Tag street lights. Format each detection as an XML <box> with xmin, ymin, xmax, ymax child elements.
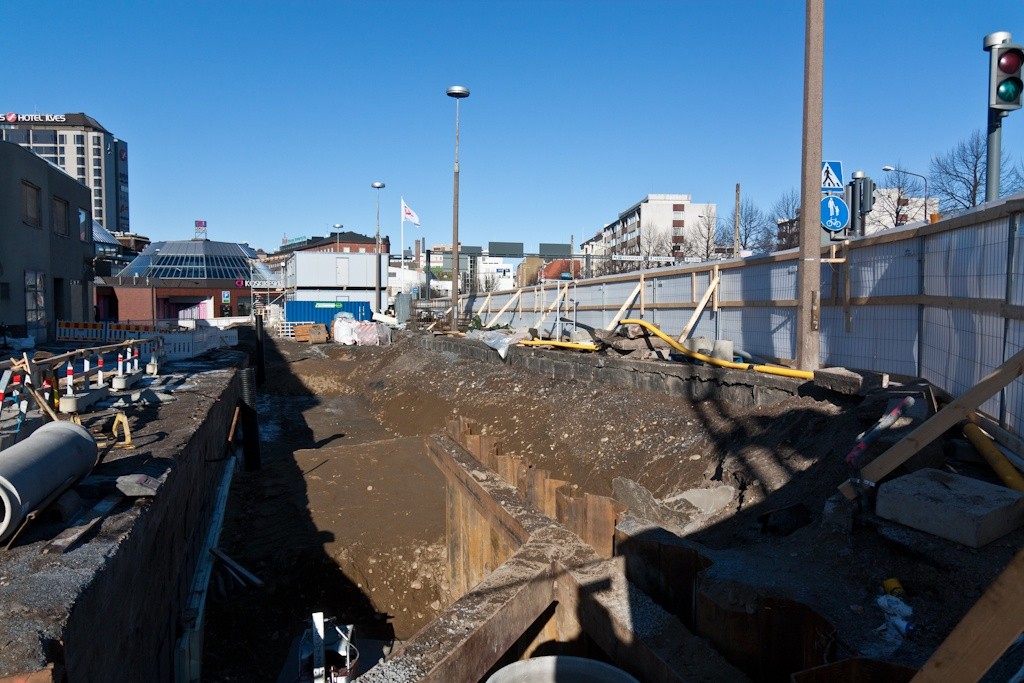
<box><xmin>333</xmin><ymin>223</ymin><xmax>344</xmax><ymax>252</ymax></box>
<box><xmin>445</xmin><ymin>86</ymin><xmax>471</xmax><ymax>333</ymax></box>
<box><xmin>372</xmin><ymin>181</ymin><xmax>385</xmax><ymax>314</ymax></box>
<box><xmin>883</xmin><ymin>166</ymin><xmax>930</xmax><ymax>222</ymax></box>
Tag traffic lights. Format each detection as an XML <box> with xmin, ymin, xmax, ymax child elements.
<box><xmin>990</xmin><ymin>45</ymin><xmax>1024</xmax><ymax>110</ymax></box>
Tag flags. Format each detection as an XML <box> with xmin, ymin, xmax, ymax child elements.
<box><xmin>403</xmin><ymin>200</ymin><xmax>421</xmax><ymax>228</ymax></box>
